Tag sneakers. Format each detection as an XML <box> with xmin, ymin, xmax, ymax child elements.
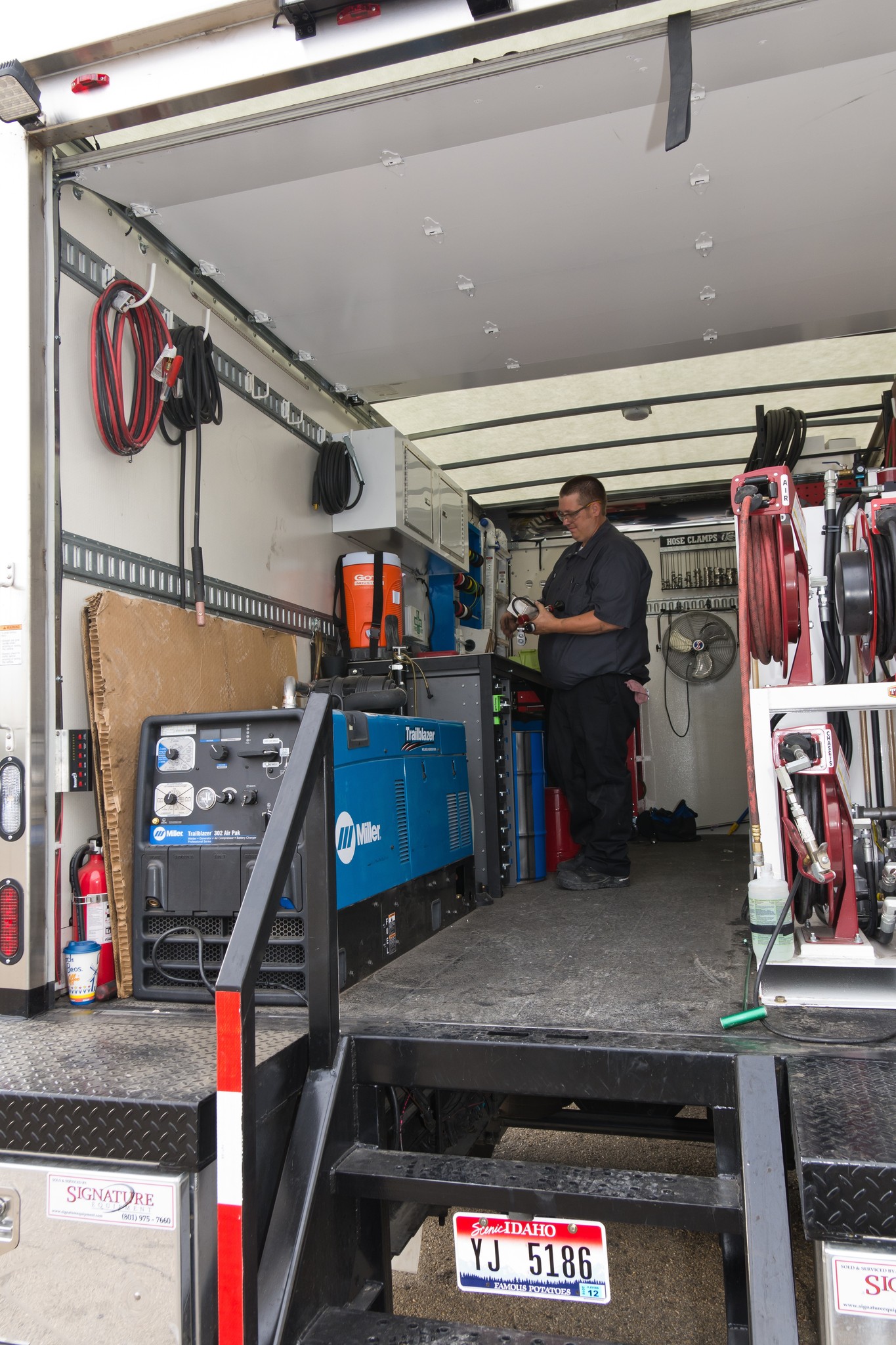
<box><xmin>556</xmin><ymin>852</ymin><xmax>586</xmax><ymax>876</ymax></box>
<box><xmin>555</xmin><ymin>863</ymin><xmax>630</xmax><ymax>891</ymax></box>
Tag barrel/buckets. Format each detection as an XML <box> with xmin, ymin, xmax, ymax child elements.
<box><xmin>334</xmin><ymin>551</ymin><xmax>403</xmax><ymax>648</ymax></box>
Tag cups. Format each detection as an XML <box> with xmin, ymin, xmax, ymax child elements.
<box><xmin>62</xmin><ymin>940</ymin><xmax>102</xmax><ymax>1006</ymax></box>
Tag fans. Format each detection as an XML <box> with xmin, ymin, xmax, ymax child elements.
<box><xmin>660</xmin><ymin>611</ymin><xmax>737</xmax><ymax>682</ymax></box>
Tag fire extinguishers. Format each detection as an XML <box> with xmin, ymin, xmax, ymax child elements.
<box><xmin>69</xmin><ymin>833</ymin><xmax>116</xmax><ymax>987</ymax></box>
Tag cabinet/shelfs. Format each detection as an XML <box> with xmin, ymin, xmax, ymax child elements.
<box><xmin>332</xmin><ymin>426</ymin><xmax>470</xmax><ymax>576</ymax></box>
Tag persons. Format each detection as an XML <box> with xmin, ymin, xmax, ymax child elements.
<box><xmin>500</xmin><ymin>474</ymin><xmax>652</xmax><ymax>890</ymax></box>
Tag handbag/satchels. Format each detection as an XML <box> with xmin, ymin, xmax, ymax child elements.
<box><xmin>636</xmin><ymin>799</ymin><xmax>699</xmax><ymax>843</ymax></box>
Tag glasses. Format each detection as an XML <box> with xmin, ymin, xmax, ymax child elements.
<box><xmin>556</xmin><ymin>501</ymin><xmax>602</xmax><ymax>523</ymax></box>
